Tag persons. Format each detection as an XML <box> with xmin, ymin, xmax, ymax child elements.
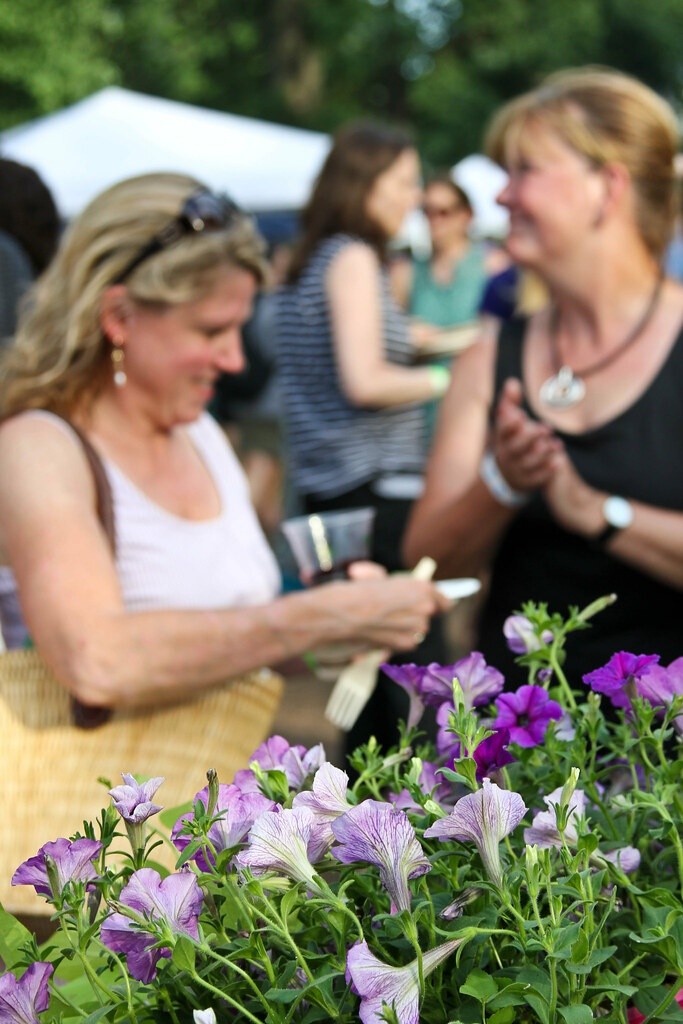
<box><xmin>0</xmin><ymin>171</ymin><xmax>454</xmax><ymax>912</ymax></box>
<box><xmin>273</xmin><ymin>117</ymin><xmax>479</xmax><ymax>576</ymax></box>
<box><xmin>409</xmin><ymin>179</ymin><xmax>505</xmax><ymax>329</ymax></box>
<box><xmin>401</xmin><ymin>63</ymin><xmax>683</xmax><ymax>787</ymax></box>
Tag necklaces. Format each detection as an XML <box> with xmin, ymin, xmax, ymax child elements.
<box><xmin>540</xmin><ymin>269</ymin><xmax>664</xmax><ymax>411</ymax></box>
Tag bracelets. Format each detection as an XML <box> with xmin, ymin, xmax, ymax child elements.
<box><xmin>481</xmin><ymin>457</ymin><xmax>524</xmax><ymax>508</ymax></box>
<box><xmin>429</xmin><ymin>363</ymin><xmax>450</xmax><ymax>399</ymax></box>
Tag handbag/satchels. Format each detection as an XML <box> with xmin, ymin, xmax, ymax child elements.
<box><xmin>0</xmin><ymin>407</ymin><xmax>283</xmax><ymax>918</ymax></box>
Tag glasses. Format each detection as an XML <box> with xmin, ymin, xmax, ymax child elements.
<box><xmin>114</xmin><ymin>189</ymin><xmax>228</xmax><ymax>285</ymax></box>
<box><xmin>421</xmin><ymin>203</ymin><xmax>465</xmax><ymax>218</ymax></box>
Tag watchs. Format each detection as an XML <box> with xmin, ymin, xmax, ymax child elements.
<box><xmin>595</xmin><ymin>497</ymin><xmax>633</xmax><ymax>544</ymax></box>
